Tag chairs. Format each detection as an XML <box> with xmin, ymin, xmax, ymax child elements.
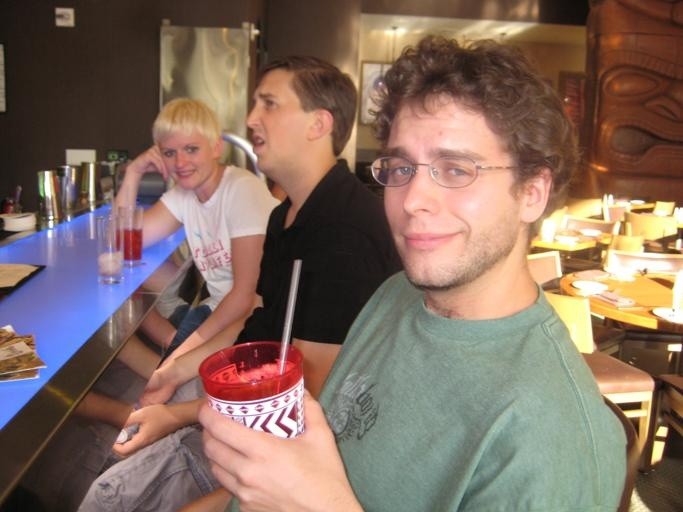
<box><xmin>529</xmin><ymin>198</ymin><xmax>683</xmax><ymax>512</ymax></box>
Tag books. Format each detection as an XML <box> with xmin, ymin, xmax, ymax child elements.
<box><xmin>0</xmin><ymin>324</ymin><xmax>48</xmax><ymax>382</ymax></box>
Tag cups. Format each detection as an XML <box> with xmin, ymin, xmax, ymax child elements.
<box><xmin>97</xmin><ymin>205</ymin><xmax>145</xmax><ymax>286</ymax></box>
<box><xmin>37</xmin><ymin>162</ymin><xmax>99</xmax><ymax>222</ymax></box>
<box><xmin>540</xmin><ymin>219</ymin><xmax>556</xmax><ymax>243</ymax></box>
<box><xmin>199</xmin><ymin>341</ymin><xmax>306</xmax><ymax>439</ymax></box>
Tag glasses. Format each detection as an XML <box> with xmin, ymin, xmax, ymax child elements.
<box><xmin>365</xmin><ymin>153</ymin><xmax>520</xmax><ymax>188</ymax></box>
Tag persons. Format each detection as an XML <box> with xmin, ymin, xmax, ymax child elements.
<box><xmin>76</xmin><ymin>54</ymin><xmax>405</xmax><ymax>511</ymax></box>
<box><xmin>107</xmin><ymin>98</ymin><xmax>279</xmax><ymax>358</ymax></box>
<box><xmin>198</xmin><ymin>35</ymin><xmax>627</xmax><ymax>510</ymax></box>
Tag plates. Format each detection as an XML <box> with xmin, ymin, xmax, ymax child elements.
<box><xmin>652</xmin><ymin>307</ymin><xmax>682</xmax><ymax>325</ymax></box>
<box><xmin>555</xmin><ymin>229</ymin><xmax>603</xmax><ymax>244</ymax></box>
<box><xmin>572</xmin><ymin>281</ymin><xmax>608</xmax><ymax>292</ymax></box>
